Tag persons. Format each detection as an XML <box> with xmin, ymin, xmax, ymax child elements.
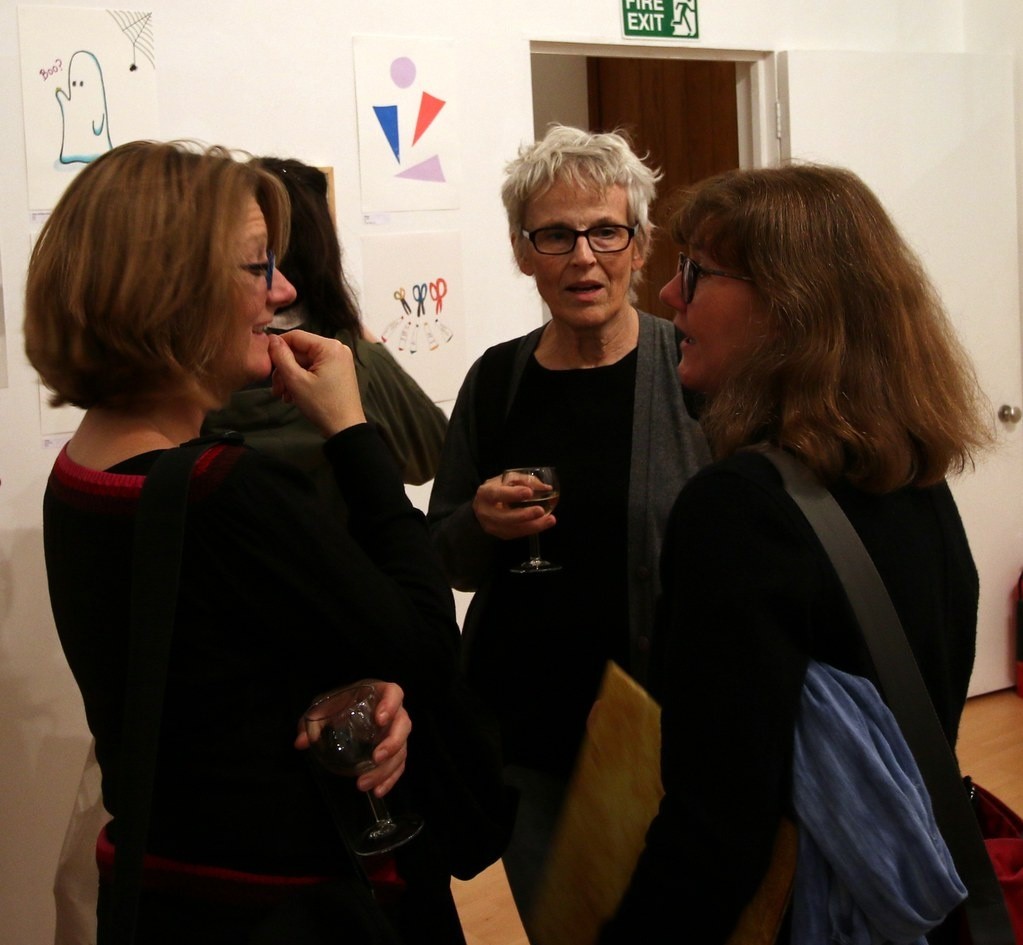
<box><xmin>24</xmin><ymin>123</ymin><xmax>1018</xmax><ymax>944</ymax></box>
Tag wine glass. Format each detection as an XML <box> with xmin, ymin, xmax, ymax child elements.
<box><xmin>304</xmin><ymin>684</ymin><xmax>424</xmax><ymax>857</ymax></box>
<box><xmin>500</xmin><ymin>465</ymin><xmax>561</xmax><ymax>573</ymax></box>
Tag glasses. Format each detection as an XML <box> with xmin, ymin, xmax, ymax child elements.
<box><xmin>676</xmin><ymin>252</ymin><xmax>758</xmax><ymax>304</ymax></box>
<box><xmin>522</xmin><ymin>223</ymin><xmax>641</xmax><ymax>257</ymax></box>
<box><xmin>238</xmin><ymin>250</ymin><xmax>279</xmax><ymax>289</ymax></box>
